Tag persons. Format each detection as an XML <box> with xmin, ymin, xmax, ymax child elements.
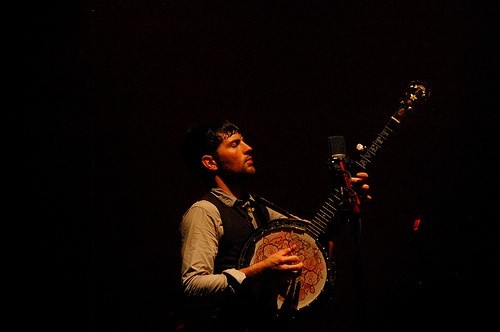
<box><xmin>180</xmin><ymin>117</ymin><xmax>372</xmax><ymax>332</ymax></box>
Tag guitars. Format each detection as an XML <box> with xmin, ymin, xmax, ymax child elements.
<box><xmin>234</xmin><ymin>81</ymin><xmax>430</xmax><ymax>310</ymax></box>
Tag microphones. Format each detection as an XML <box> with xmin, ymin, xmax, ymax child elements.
<box><xmin>327</xmin><ymin>135</ymin><xmax>361</xmax><ymax>212</ymax></box>
